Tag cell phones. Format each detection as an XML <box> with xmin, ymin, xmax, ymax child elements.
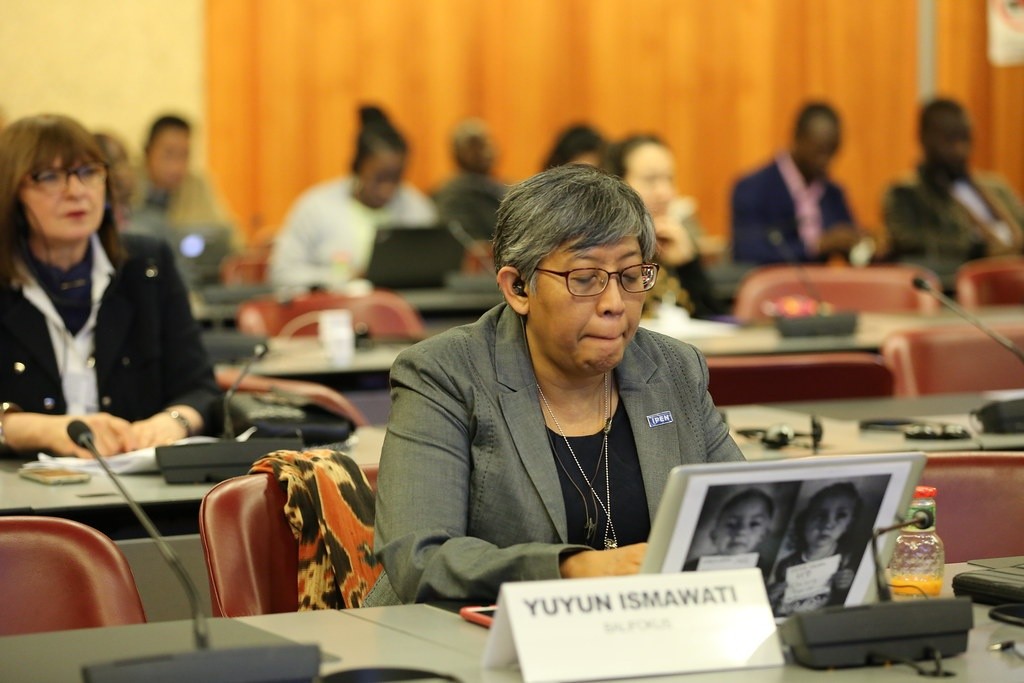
<box><xmin>460</xmin><ymin>605</ymin><xmax>496</xmax><ymax>628</ymax></box>
<box><xmin>860</xmin><ymin>418</ymin><xmax>913</xmax><ymax>429</ymax></box>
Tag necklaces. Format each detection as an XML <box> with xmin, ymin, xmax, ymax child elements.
<box><xmin>534</xmin><ymin>370</ymin><xmax>620</xmax><ymax>550</ymax></box>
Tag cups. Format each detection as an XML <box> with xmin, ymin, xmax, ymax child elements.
<box><xmin>315</xmin><ymin>311</ymin><xmax>354</xmax><ymax>360</ymax></box>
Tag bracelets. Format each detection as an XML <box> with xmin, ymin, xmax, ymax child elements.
<box><xmin>162</xmin><ymin>407</ymin><xmax>192</xmax><ymax>438</ymax></box>
<box><xmin>0</xmin><ymin>401</ymin><xmax>24</xmax><ymax>446</ymax></box>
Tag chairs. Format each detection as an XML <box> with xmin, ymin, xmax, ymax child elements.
<box><xmin>0</xmin><ymin>259</ymin><xmax>1024</xmax><ymax>634</ymax></box>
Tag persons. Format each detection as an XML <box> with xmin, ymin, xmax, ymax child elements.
<box><xmin>427</xmin><ymin>117</ymin><xmax>508</xmax><ymax>239</ymax></box>
<box><xmin>767</xmin><ymin>481</ymin><xmax>859</xmax><ymax>617</ymax></box>
<box><xmin>0</xmin><ymin>113</ymin><xmax>232</xmax><ymax>460</ymax></box>
<box><xmin>682</xmin><ymin>487</ymin><xmax>775</xmax><ymax>571</ymax></box>
<box><xmin>263</xmin><ymin>104</ymin><xmax>442</xmax><ymax>302</ymax></box>
<box><xmin>544</xmin><ymin>123</ymin><xmax>735</xmax><ymax>320</ymax></box>
<box><xmin>362</xmin><ymin>162</ymin><xmax>752</xmax><ymax>612</ymax></box>
<box><xmin>732</xmin><ymin>101</ymin><xmax>861</xmax><ymax>264</ymax></box>
<box><xmin>885</xmin><ymin>98</ymin><xmax>1024</xmax><ymax>292</ymax></box>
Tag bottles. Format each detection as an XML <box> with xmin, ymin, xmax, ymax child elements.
<box><xmin>890</xmin><ymin>485</ymin><xmax>944</xmax><ymax>597</ymax></box>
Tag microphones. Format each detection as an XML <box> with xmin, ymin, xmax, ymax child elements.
<box><xmin>154</xmin><ymin>343</ymin><xmax>303</xmax><ymax>486</ymax></box>
<box><xmin>779</xmin><ymin>508</ymin><xmax>973</xmax><ymax>669</ymax></box>
<box><xmin>66</xmin><ymin>419</ymin><xmax>320</xmax><ymax>683</ymax></box>
<box><xmin>911</xmin><ymin>275</ymin><xmax>1024</xmax><ymax>366</ymax></box>
<box><xmin>766</xmin><ymin>230</ymin><xmax>856</xmax><ymax>339</ymax></box>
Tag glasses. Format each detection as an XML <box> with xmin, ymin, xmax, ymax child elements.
<box><xmin>26</xmin><ymin>161</ymin><xmax>106</xmax><ymax>196</ymax></box>
<box><xmin>536</xmin><ymin>263</ymin><xmax>661</xmax><ymax>297</ymax></box>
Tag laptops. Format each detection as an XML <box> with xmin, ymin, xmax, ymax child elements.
<box><xmin>638</xmin><ymin>452</ymin><xmax>927</xmax><ymax>627</ymax></box>
<box><xmin>365</xmin><ymin>226</ymin><xmax>470</xmax><ymax>291</ymax></box>
<box><xmin>153</xmin><ymin>225</ymin><xmax>232</xmax><ymax>287</ymax></box>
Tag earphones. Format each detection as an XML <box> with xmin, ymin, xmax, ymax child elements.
<box><xmin>511</xmin><ymin>276</ymin><xmax>528</xmax><ymax>299</ymax></box>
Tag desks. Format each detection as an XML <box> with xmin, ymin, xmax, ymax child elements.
<box><xmin>0</xmin><ymin>278</ymin><xmax>1024</xmax><ymax>683</ymax></box>
